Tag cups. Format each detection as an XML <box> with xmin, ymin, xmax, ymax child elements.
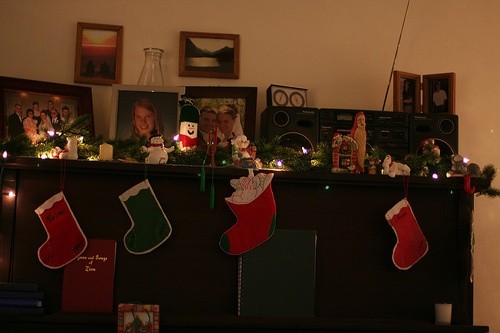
<box><xmin>434</xmin><ymin>303</ymin><xmax>452</xmax><ymax>326</ymax></box>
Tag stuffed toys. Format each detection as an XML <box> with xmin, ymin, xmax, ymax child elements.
<box><xmin>140</xmin><ymin>130</ymin><xmax>175</xmax><ymax>164</ymax></box>
<box><xmin>230</xmin><ymin>135</ymin><xmax>257</xmax><ymax>164</ymax></box>
<box><xmin>380</xmin><ymin>152</ymin><xmax>411</xmax><ymax>178</ymax></box>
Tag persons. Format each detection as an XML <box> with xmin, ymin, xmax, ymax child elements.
<box><xmin>199</xmin><ymin>106</ymin><xmax>220</xmax><ymax>144</ymax></box>
<box><xmin>217</xmin><ymin>104</ymin><xmax>238</xmax><ymax>141</ymax></box>
<box><xmin>8</xmin><ymin>100</ymin><xmax>69</xmax><ymax>138</ymax></box>
<box><xmin>128</xmin><ymin>97</ymin><xmax>165</xmax><ymax>141</ymax></box>
<box><xmin>403</xmin><ymin>82</ymin><xmax>413</xmax><ymax>112</ymax></box>
<box><xmin>433</xmin><ymin>83</ymin><xmax>447</xmax><ymax>112</ymax></box>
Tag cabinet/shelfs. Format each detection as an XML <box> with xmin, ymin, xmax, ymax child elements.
<box><xmin>0</xmin><ymin>155</ymin><xmax>489</xmax><ymax>333</ymax></box>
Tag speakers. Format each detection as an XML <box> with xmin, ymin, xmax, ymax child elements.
<box><xmin>261</xmin><ymin>106</ymin><xmax>319</xmax><ymax>155</ymax></box>
<box><xmin>409</xmin><ymin>113</ymin><xmax>459</xmax><ymax>160</ymax></box>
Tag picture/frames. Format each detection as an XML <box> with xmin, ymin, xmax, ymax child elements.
<box><xmin>185</xmin><ymin>86</ymin><xmax>257</xmax><ymax>142</ymax></box>
<box><xmin>422</xmin><ymin>72</ymin><xmax>456</xmax><ymax>115</ymax></box>
<box><xmin>118</xmin><ymin>304</ymin><xmax>160</xmax><ymax>333</ymax></box>
<box><xmin>179</xmin><ymin>31</ymin><xmax>240</xmax><ymax>79</ymax></box>
<box><xmin>0</xmin><ymin>76</ymin><xmax>96</xmax><ymax>140</ymax></box>
<box><xmin>109</xmin><ymin>84</ymin><xmax>186</xmax><ymax>140</ymax></box>
<box><xmin>393</xmin><ymin>70</ymin><xmax>422</xmax><ymax>113</ymax></box>
<box><xmin>74</xmin><ymin>22</ymin><xmax>124</xmax><ymax>84</ymax></box>
